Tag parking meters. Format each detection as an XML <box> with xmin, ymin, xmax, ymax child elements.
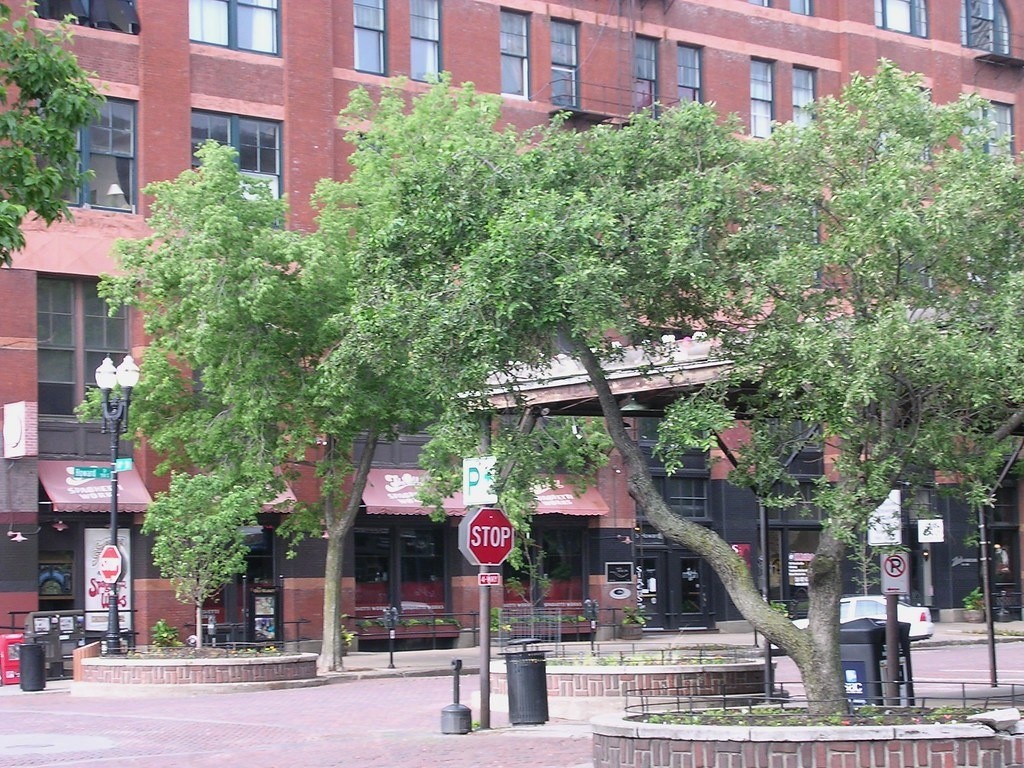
<box><xmin>585</xmin><ymin>598</ymin><xmax>601</xmax><ymax>656</ymax></box>
<box><xmin>384</xmin><ymin>608</ymin><xmax>399</xmax><ymax>669</ymax></box>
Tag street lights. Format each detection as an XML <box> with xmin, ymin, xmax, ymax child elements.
<box><xmin>96</xmin><ymin>354</ymin><xmax>140</xmax><ymax>659</ymax></box>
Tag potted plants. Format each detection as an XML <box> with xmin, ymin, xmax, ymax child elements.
<box><xmin>506</xmin><ymin>615</ymin><xmax>598</xmax><ymax>634</ymax></box>
<box><xmin>618</xmin><ymin>605</ymin><xmax>646</xmax><ymax>640</ymax></box>
<box><xmin>961</xmin><ymin>586</ymin><xmax>988</xmax><ymax>625</ymax></box>
<box><xmin>356</xmin><ymin>617</ymin><xmax>461</xmax><ymax>640</ymax></box>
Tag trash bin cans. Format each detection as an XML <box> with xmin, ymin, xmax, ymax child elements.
<box><xmin>496</xmin><ymin>638</ymin><xmax>554</xmax><ymax>725</ymax></box>
<box><xmin>18</xmin><ymin>634</ymin><xmax>46</xmax><ymax>692</ymax></box>
<box><xmin>840</xmin><ymin>618</ymin><xmax>916</xmax><ymax>714</ymax></box>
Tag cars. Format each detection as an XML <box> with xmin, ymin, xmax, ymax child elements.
<box><xmin>791</xmin><ymin>595</ymin><xmax>935</xmax><ymax>644</ymax></box>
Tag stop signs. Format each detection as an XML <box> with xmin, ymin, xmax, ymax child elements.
<box><xmin>98</xmin><ymin>543</ymin><xmax>123</xmax><ymax>585</ymax></box>
<box><xmin>467</xmin><ymin>507</ymin><xmax>515</xmax><ymax>566</ymax></box>
